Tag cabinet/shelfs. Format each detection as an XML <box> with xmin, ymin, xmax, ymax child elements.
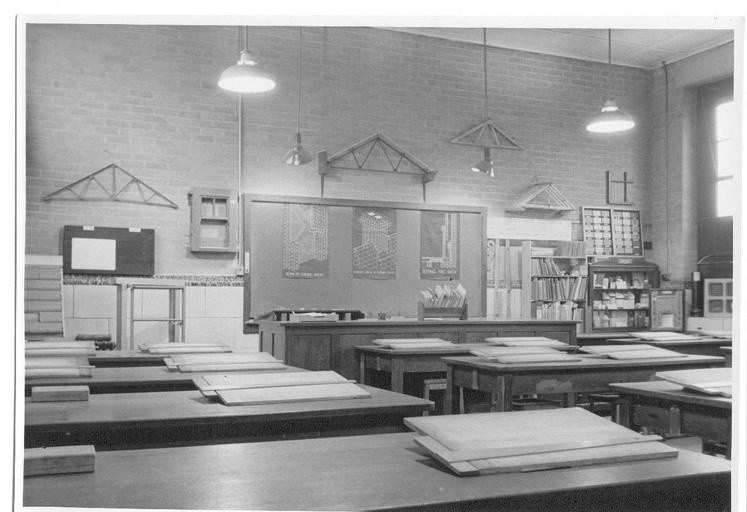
<box><xmin>487</xmin><ymin>237</ymin><xmax>661</xmax><ymax>335</ymax></box>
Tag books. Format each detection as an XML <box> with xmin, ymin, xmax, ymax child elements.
<box><xmin>530</xmin><ymin>237</ymin><xmax>587</xmax><ymax>335</ymax></box>
<box><xmin>418</xmin><ymin>283</ymin><xmax>468</xmax><ymax>309</ymax></box>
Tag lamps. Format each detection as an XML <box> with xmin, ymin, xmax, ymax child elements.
<box><xmin>586</xmin><ymin>29</ymin><xmax>636</xmax><ymax>133</ymax></box>
<box><xmin>218</xmin><ymin>26</ymin><xmax>276</xmax><ymax>94</ymax></box>
<box><xmin>470</xmin><ymin>27</ymin><xmax>496</xmax><ymax>178</ymax></box>
<box><xmin>283</xmin><ymin>27</ymin><xmax>314</xmax><ymax>165</ymax></box>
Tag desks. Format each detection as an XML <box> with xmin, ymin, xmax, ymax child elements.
<box><xmin>24</xmin><ymin>321</ymin><xmax>731</xmax><ymax>512</ymax></box>
